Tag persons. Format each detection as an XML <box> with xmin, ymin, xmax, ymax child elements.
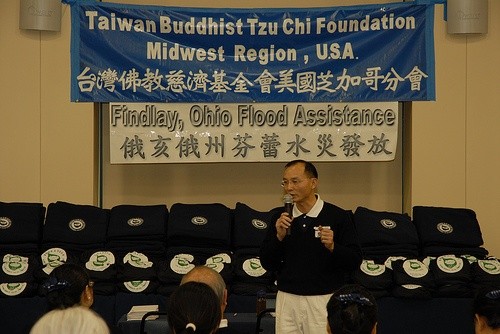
<box><xmin>260</xmin><ymin>159</ymin><xmax>362</xmax><ymax>334</ymax></box>
<box><xmin>32</xmin><ymin>265</ymin><xmax>228</xmax><ymax>334</ymax></box>
<box><xmin>472</xmin><ymin>279</ymin><xmax>500</xmax><ymax>334</ymax></box>
<box><xmin>326</xmin><ymin>284</ymin><xmax>379</xmax><ymax>334</ymax></box>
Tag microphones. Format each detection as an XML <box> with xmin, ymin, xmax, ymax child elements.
<box><xmin>283</xmin><ymin>194</ymin><xmax>293</xmax><ymax>235</ymax></box>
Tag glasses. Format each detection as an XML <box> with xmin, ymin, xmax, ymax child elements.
<box><xmin>280</xmin><ymin>177</ymin><xmax>314</xmax><ymax>187</ymax></box>
<box><xmin>89</xmin><ymin>281</ymin><xmax>94</xmax><ymax>287</ymax></box>
